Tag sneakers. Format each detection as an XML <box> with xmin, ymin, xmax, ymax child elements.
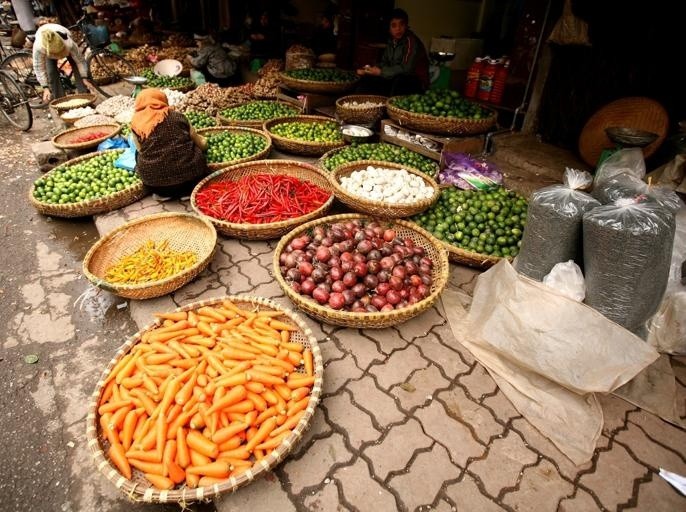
<box><xmin>151</xmin><ymin>194</ymin><xmax>173</xmax><ymax>202</ymax></box>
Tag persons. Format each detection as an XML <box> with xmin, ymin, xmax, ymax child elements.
<box><xmin>225</xmin><ymin>8</ymin><xmax>273</xmax><ymax>67</ymax></box>
<box><xmin>320</xmin><ymin>10</ymin><xmax>370</xmax><ymax>68</ymax></box>
<box><xmin>186</xmin><ymin>27</ymin><xmax>238</xmax><ymax>84</ymax></box>
<box><xmin>358</xmin><ymin>8</ymin><xmax>430</xmax><ymax>96</ymax></box>
<box><xmin>129</xmin><ymin>88</ymin><xmax>209</xmax><ymax>202</ymax></box>
<box><xmin>32</xmin><ymin>23</ymin><xmax>94</xmax><ymax>102</ymax></box>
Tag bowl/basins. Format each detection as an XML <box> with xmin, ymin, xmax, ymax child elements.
<box><xmin>338</xmin><ymin>124</ymin><xmax>375</xmax><ymax>143</ymax></box>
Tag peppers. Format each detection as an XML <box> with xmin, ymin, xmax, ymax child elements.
<box><xmin>194</xmin><ymin>174</ymin><xmax>331</xmax><ymax>224</ymax></box>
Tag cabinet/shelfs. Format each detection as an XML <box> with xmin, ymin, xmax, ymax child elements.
<box><xmin>378</xmin><ymin>119</ymin><xmax>485</xmax><ymax>171</ymax></box>
<box><xmin>276</xmin><ymin>83</ymin><xmax>334</xmax><ymax>113</ymax></box>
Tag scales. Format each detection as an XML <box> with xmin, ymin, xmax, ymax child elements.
<box><xmin>429</xmin><ymin>52</ymin><xmax>456</xmax><ymax>89</ymax></box>
<box><xmin>592</xmin><ymin>127</ymin><xmax>659</xmax><ymax>178</ymax></box>
<box><xmin>123</xmin><ymin>76</ymin><xmax>147</xmax><ymax>98</ymax></box>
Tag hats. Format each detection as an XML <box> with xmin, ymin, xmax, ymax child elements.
<box><xmin>36</xmin><ymin>28</ymin><xmax>70</xmax><ymax>59</ymax></box>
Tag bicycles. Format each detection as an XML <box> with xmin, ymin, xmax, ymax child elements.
<box><xmin>0</xmin><ymin>8</ymin><xmax>136</xmax><ymax>109</ymax></box>
<box><xmin>1</xmin><ymin>43</ymin><xmax>33</xmax><ymax>131</ymax></box>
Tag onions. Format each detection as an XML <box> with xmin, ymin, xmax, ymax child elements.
<box><xmin>281</xmin><ymin>219</ymin><xmax>432</xmax><ymax>311</ymax></box>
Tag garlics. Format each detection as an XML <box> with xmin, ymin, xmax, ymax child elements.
<box><xmin>343</xmin><ymin>100</ymin><xmax>384</xmax><ymax>111</ymax></box>
<box><xmin>339</xmin><ymin>165</ymin><xmax>435</xmax><ymax>203</ymax></box>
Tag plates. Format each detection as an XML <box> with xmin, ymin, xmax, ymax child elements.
<box><xmin>154</xmin><ymin>58</ymin><xmax>183</xmax><ymax>78</ymax></box>
<box><xmin>123</xmin><ymin>76</ymin><xmax>147</xmax><ymax>85</ymax></box>
<box><xmin>605</xmin><ymin>126</ymin><xmax>659</xmax><ymax>146</ymax></box>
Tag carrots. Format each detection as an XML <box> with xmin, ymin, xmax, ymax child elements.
<box><xmin>97</xmin><ymin>300</ymin><xmax>315</xmax><ymax>491</ymax></box>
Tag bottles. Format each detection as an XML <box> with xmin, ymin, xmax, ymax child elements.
<box><xmin>465</xmin><ymin>55</ymin><xmax>511</xmax><ymax>103</ymax></box>
